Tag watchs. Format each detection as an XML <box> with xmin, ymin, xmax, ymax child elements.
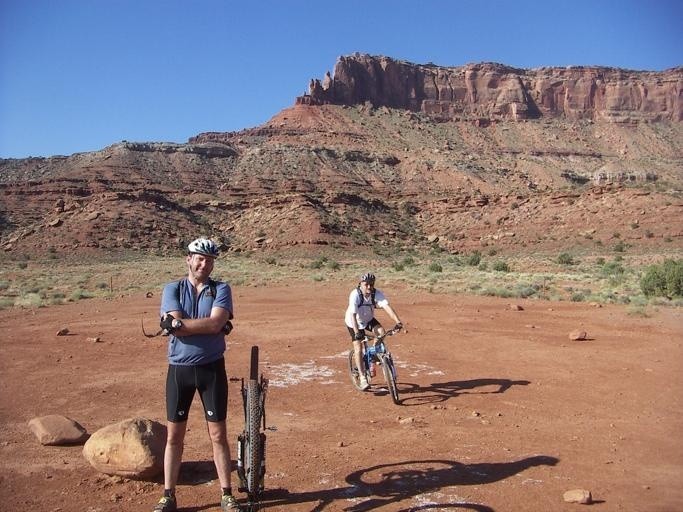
<box><xmin>174</xmin><ymin>319</ymin><xmax>182</xmax><ymax>331</ymax></box>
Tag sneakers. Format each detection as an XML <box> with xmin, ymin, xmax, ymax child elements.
<box><xmin>153</xmin><ymin>496</ymin><xmax>176</xmax><ymax>512</ymax></box>
<box><xmin>360</xmin><ymin>375</ymin><xmax>370</xmax><ymax>389</ymax></box>
<box><xmin>221</xmin><ymin>494</ymin><xmax>244</xmax><ymax>512</ymax></box>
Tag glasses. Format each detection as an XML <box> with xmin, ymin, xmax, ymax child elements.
<box><xmin>141</xmin><ymin>313</ymin><xmax>164</xmax><ymax>338</ymax></box>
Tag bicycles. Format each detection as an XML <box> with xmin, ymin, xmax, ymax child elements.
<box><xmin>230</xmin><ymin>343</ymin><xmax>271</xmax><ymax>508</ymax></box>
<box><xmin>347</xmin><ymin>320</ymin><xmax>407</xmax><ymax>407</ymax></box>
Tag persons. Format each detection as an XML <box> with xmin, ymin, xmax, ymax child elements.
<box><xmin>153</xmin><ymin>238</ymin><xmax>240</xmax><ymax>510</ymax></box>
<box><xmin>345</xmin><ymin>272</ymin><xmax>407</xmax><ymax>391</ymax></box>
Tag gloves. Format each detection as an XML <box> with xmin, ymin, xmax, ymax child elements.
<box><xmin>395</xmin><ymin>322</ymin><xmax>402</xmax><ymax>329</ymax></box>
<box><xmin>355</xmin><ymin>331</ymin><xmax>364</xmax><ymax>339</ymax></box>
<box><xmin>160</xmin><ymin>314</ymin><xmax>174</xmax><ymax>330</ymax></box>
<box><xmin>221</xmin><ymin>321</ymin><xmax>233</xmax><ymax>335</ymax></box>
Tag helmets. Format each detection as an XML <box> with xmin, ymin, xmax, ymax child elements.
<box><xmin>361</xmin><ymin>273</ymin><xmax>375</xmax><ymax>282</ymax></box>
<box><xmin>188</xmin><ymin>238</ymin><xmax>219</xmax><ymax>259</ymax></box>
